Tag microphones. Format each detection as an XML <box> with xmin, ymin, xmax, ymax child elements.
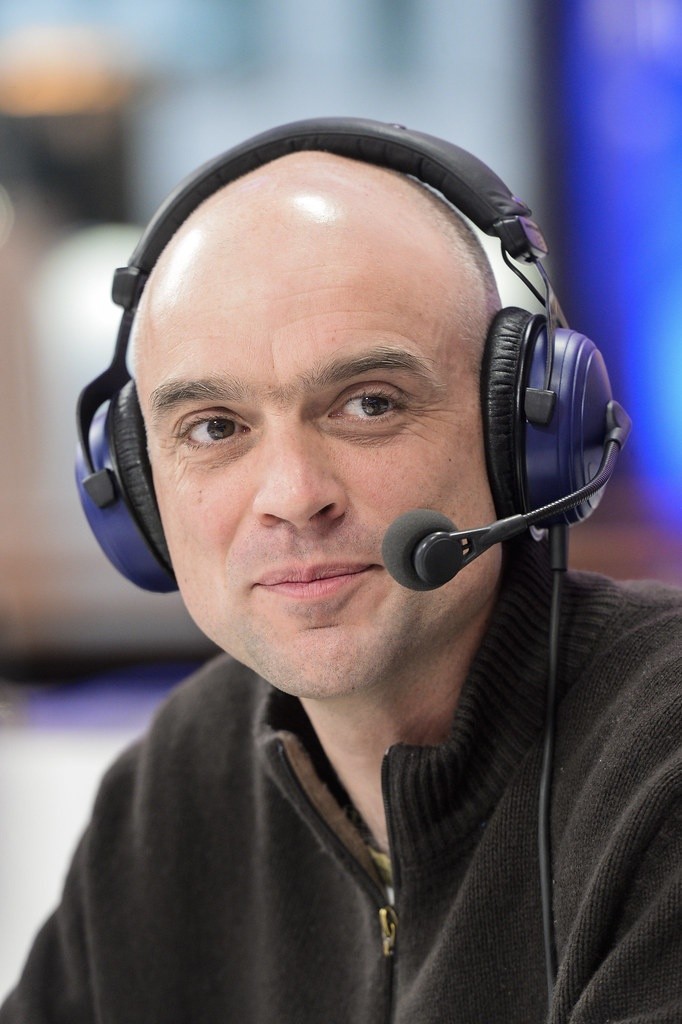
<box><xmin>382</xmin><ymin>400</ymin><xmax>631</xmax><ymax>591</ymax></box>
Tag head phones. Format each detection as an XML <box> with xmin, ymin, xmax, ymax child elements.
<box><xmin>72</xmin><ymin>118</ymin><xmax>632</xmax><ymax>594</ymax></box>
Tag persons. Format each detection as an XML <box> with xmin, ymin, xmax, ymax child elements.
<box><xmin>1</xmin><ymin>150</ymin><xmax>682</xmax><ymax>1024</ymax></box>
